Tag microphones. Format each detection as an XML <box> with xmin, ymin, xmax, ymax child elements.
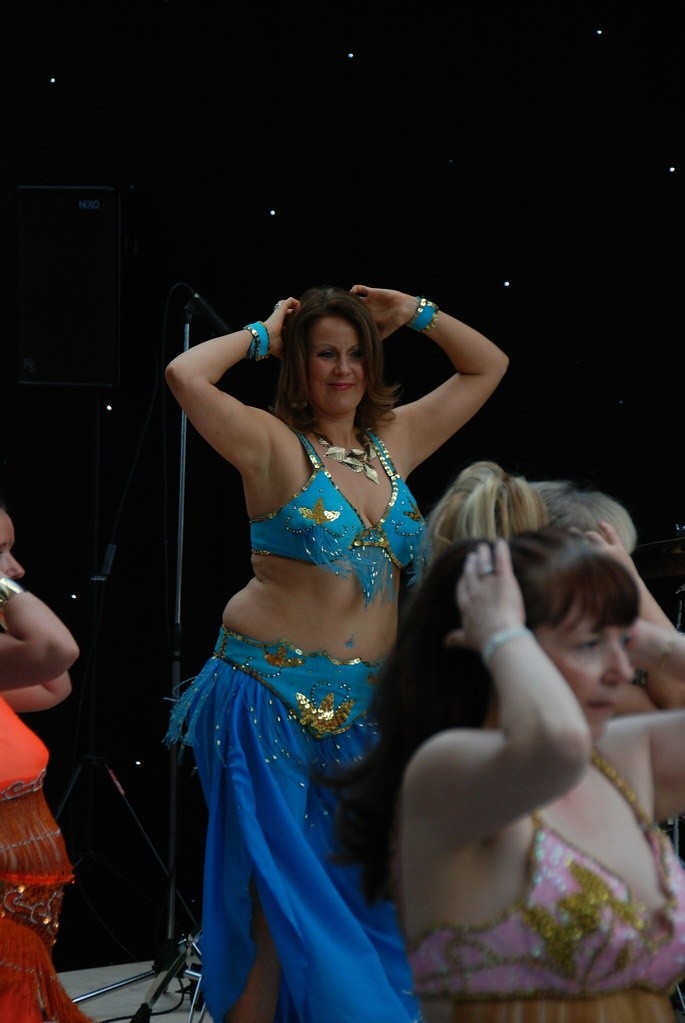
<box><xmin>188</xmin><ymin>286</ymin><xmax>234</xmax><ymax>334</ymax></box>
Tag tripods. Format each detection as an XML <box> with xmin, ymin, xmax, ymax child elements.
<box><xmin>52</xmin><ymin>391</ymin><xmax>202</xmax><ymax>1004</ymax></box>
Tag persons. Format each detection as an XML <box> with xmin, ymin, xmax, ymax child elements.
<box><xmin>165</xmin><ymin>284</ymin><xmax>509</xmax><ymax>1023</ymax></box>
<box><xmin>0</xmin><ymin>503</ymin><xmax>97</xmax><ymax>1022</ymax></box>
<box><xmin>307</xmin><ymin>461</ymin><xmax>685</xmax><ymax>1023</ymax></box>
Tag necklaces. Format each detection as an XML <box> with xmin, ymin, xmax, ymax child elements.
<box><xmin>312</xmin><ymin>429</ymin><xmax>380</xmax><ymax>485</ymax></box>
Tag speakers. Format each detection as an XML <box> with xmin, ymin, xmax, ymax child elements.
<box><xmin>15</xmin><ymin>182</ymin><xmax>121</xmax><ymax>392</ymax></box>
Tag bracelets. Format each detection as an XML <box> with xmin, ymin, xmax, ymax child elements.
<box><xmin>243</xmin><ymin>320</ymin><xmax>270</xmax><ymax>361</ymax></box>
<box><xmin>405</xmin><ymin>296</ymin><xmax>439</xmax><ymax>332</ymax></box>
<box><xmin>0</xmin><ymin>578</ymin><xmax>25</xmax><ymax>609</ymax></box>
<box><xmin>482</xmin><ymin>628</ymin><xmax>533</xmax><ymax>666</ymax></box>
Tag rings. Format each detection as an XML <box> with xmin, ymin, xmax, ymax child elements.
<box><xmin>275</xmin><ymin>304</ymin><xmax>280</xmax><ymax>309</ymax></box>
<box><xmin>478</xmin><ymin>564</ymin><xmax>495</xmax><ymax>577</ymax></box>
